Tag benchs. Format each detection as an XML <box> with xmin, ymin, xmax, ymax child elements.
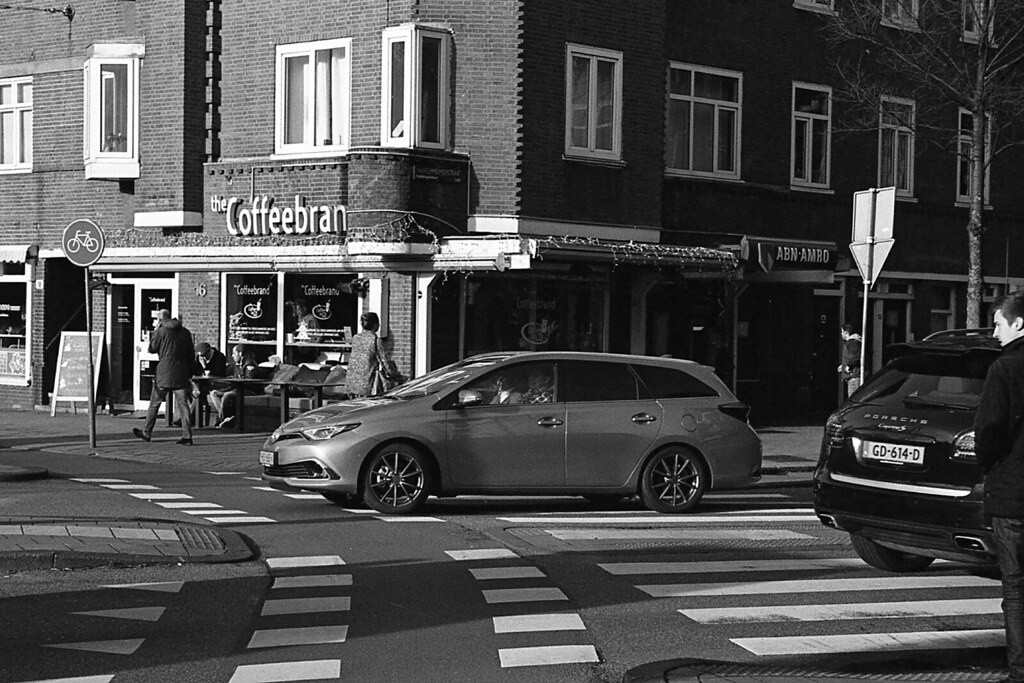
<box><xmin>226</xmin><ymin>362</ymin><xmax>332</xmax><ymax>400</ymax></box>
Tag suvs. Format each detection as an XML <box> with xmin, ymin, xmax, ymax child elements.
<box><xmin>812</xmin><ymin>326</ymin><xmax>999</xmax><ymax>573</ymax></box>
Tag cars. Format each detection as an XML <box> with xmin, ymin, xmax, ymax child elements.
<box><xmin>259</xmin><ymin>348</ymin><xmax>763</xmax><ymax>516</ymax></box>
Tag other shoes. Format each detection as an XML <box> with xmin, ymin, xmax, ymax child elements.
<box><xmin>215</xmin><ymin>416</ymin><xmax>236</xmax><ymax>429</ymax></box>
<box><xmin>133</xmin><ymin>428</ymin><xmax>151</xmax><ymax>442</ymax></box>
<box><xmin>176</xmin><ymin>437</ymin><xmax>193</xmax><ymax>444</ymax></box>
<box><xmin>173</xmin><ymin>418</ymin><xmax>182</xmax><ymax>427</ymax></box>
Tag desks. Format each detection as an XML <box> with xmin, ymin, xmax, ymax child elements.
<box><xmin>139</xmin><ymin>374</ymin><xmax>345</xmax><ymax>436</ymax></box>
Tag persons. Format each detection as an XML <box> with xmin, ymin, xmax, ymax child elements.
<box><xmin>343</xmin><ymin>311</ymin><xmax>402</xmax><ymax>401</ymax></box>
<box><xmin>836</xmin><ymin>323</ymin><xmax>861</xmax><ymax>397</ymax></box>
<box><xmin>971</xmin><ymin>289</ymin><xmax>1024</xmax><ymax>683</ymax></box>
<box><xmin>490</xmin><ymin>369</ymin><xmax>525</xmax><ymax>406</ymax></box>
<box><xmin>132</xmin><ymin>309</ymin><xmax>196</xmax><ymax>445</ymax></box>
<box><xmin>210</xmin><ymin>345</ymin><xmax>264</xmax><ymax>433</ymax></box>
<box><xmin>170</xmin><ymin>342</ymin><xmax>228</xmax><ymax>427</ymax></box>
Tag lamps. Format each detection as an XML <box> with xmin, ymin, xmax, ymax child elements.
<box><xmin>336</xmin><ymin>276</ymin><xmax>369</xmax><ymax>300</ymax></box>
<box><xmin>88</xmin><ymin>276</ymin><xmax>112</xmax><ymax>292</ymax></box>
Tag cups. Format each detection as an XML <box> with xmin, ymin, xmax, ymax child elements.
<box><xmin>204</xmin><ymin>370</ymin><xmax>211</xmax><ymax>376</ymax></box>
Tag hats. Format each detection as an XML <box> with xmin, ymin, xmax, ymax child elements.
<box><xmin>195</xmin><ymin>343</ymin><xmax>211</xmax><ymax>357</ymax></box>
